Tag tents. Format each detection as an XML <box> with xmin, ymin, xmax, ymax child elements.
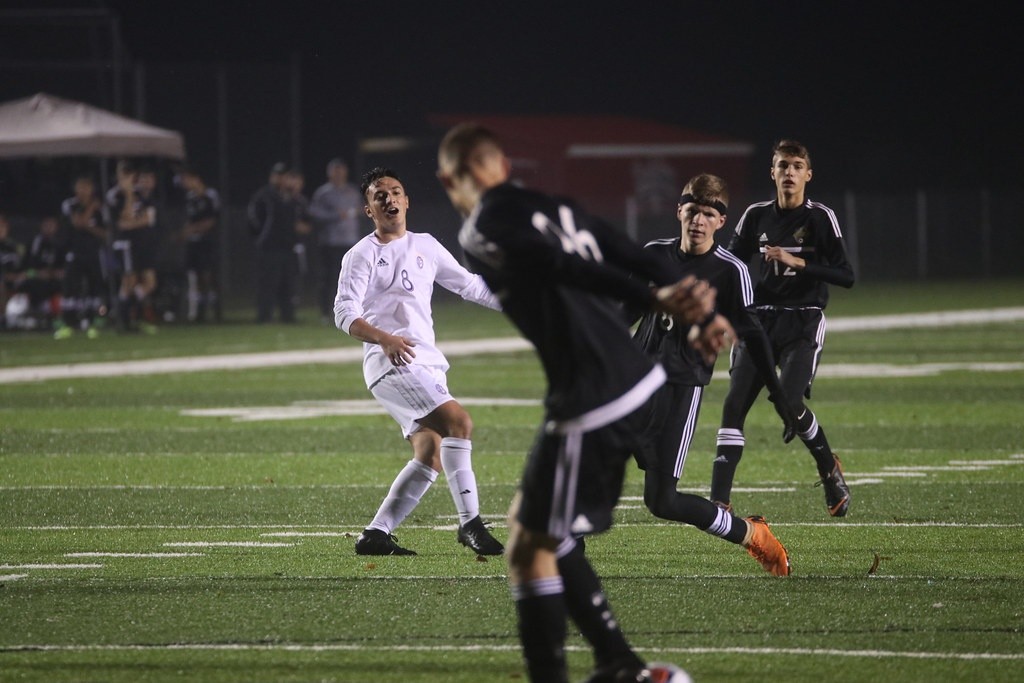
<box><xmin>0</xmin><ymin>94</ymin><xmax>186</xmax><ymax>208</ymax></box>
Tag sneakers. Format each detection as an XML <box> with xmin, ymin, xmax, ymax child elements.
<box><xmin>743</xmin><ymin>516</ymin><xmax>790</xmax><ymax>577</ymax></box>
<box><xmin>457</xmin><ymin>515</ymin><xmax>504</xmax><ymax>556</ymax></box>
<box><xmin>813</xmin><ymin>453</ymin><xmax>851</xmax><ymax>516</ymax></box>
<box><xmin>355</xmin><ymin>528</ymin><xmax>417</xmax><ymax>556</ymax></box>
<box><xmin>710</xmin><ymin>500</ymin><xmax>734</xmax><ymax>517</ymax></box>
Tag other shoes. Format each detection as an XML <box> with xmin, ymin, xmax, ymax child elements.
<box><xmin>87</xmin><ymin>328</ymin><xmax>99</xmax><ymax>339</ymax></box>
<box><xmin>635</xmin><ymin>663</ymin><xmax>689</xmax><ymax>683</ymax></box>
<box><xmin>142</xmin><ymin>323</ymin><xmax>156</xmax><ymax>334</ymax></box>
<box><xmin>53</xmin><ymin>327</ymin><xmax>71</xmax><ymax>340</ymax></box>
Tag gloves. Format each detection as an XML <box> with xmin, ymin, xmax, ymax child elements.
<box><xmin>764</xmin><ymin>371</ymin><xmax>797</xmax><ymax>443</ymax></box>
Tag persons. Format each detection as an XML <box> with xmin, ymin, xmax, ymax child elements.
<box><xmin>3</xmin><ymin>125</ymin><xmax>860</xmax><ymax>576</ymax></box>
<box><xmin>438</xmin><ymin>124</ymin><xmax>721</xmax><ymax>683</ymax></box>
